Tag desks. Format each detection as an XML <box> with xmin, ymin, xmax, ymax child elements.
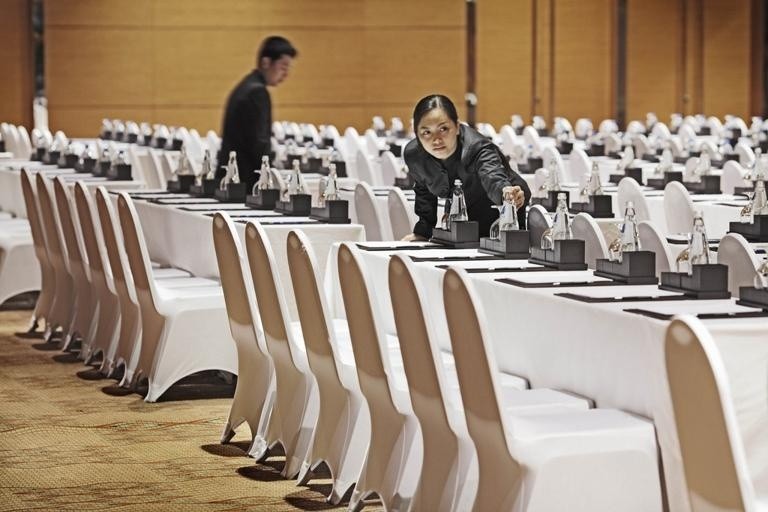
<box><xmin>105</xmin><ymin>189</ymin><xmax>364</xmax><ymax>321</ymax></box>
<box><xmin>332</xmin><ymin>242</ymin><xmax>766</xmax><ymax>512</ymax></box>
<box><xmin>65</xmin><ymin>138</ymin><xmax>183</xmax><ymax>157</ymax></box>
<box><xmin>1</xmin><ymin>151</ymin><xmax>143</xmax><ymax>186</ymax></box>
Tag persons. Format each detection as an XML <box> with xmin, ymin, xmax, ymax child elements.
<box><xmin>216</xmin><ymin>36</ymin><xmax>298</xmax><ymax>193</ymax></box>
<box><xmin>403</xmin><ymin>95</ymin><xmax>531</xmax><ymax>241</ymax></box>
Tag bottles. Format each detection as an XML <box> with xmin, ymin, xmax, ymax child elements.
<box><xmin>607</xmin><ymin>200</ymin><xmax>639</xmax><ymax>263</ymax></box>
<box><xmin>488</xmin><ymin>186</ymin><xmax>518</xmax><ymax>240</ymax></box>
<box><xmin>79</xmin><ymin>144</ymin><xmax>91</xmax><ymax>164</ymax></box>
<box><xmin>110</xmin><ymin>150</ymin><xmax>125</xmax><ymax>170</ymax></box>
<box><xmin>94</xmin><ymin>147</ymin><xmax>108</xmax><ymax>169</ymax></box>
<box><xmin>281</xmin><ymin>160</ymin><xmax>304</xmax><ymax>203</ymax></box>
<box><xmin>537</xmin><ymin>158</ymin><xmax>560</xmax><ymax>191</ymax></box>
<box><xmin>579</xmin><ymin>163</ymin><xmax>601</xmax><ymax>196</ymax></box>
<box><xmin>194</xmin><ymin>149</ymin><xmax>214</xmax><ymax>186</ymax></box>
<box><xmin>653</xmin><ymin>146</ymin><xmax>672</xmax><ymax>179</ymax></box>
<box><xmin>440</xmin><ymin>179</ymin><xmax>467</xmax><ymax>230</ymax></box>
<box><xmin>317</xmin><ymin>164</ymin><xmax>341</xmax><ymax>208</ymax></box>
<box><xmin>219</xmin><ymin>150</ymin><xmax>239</xmax><ymax>190</ymax></box>
<box><xmin>539</xmin><ymin>193</ymin><xmax>572</xmax><ymax>252</ymax></box>
<box><xmin>688</xmin><ymin>149</ymin><xmax>710</xmax><ymax>182</ymax></box>
<box><xmin>743</xmin><ymin>147</ymin><xmax>764</xmax><ymax>179</ymax></box>
<box><xmin>252</xmin><ymin>155</ymin><xmax>273</xmax><ymax>196</ymax></box>
<box><xmin>739</xmin><ymin>173</ymin><xmax>767</xmax><ymax>216</ymax></box>
<box><xmin>616</xmin><ymin>140</ymin><xmax>634</xmax><ymax>175</ymax></box>
<box><xmin>674</xmin><ymin>217</ymin><xmax>709</xmax><ymax>277</ymax></box>
<box><xmin>59</xmin><ymin>141</ymin><xmax>71</xmax><ymax>164</ymax></box>
<box><xmin>168</xmin><ymin>146</ymin><xmax>192</xmax><ymax>182</ymax></box>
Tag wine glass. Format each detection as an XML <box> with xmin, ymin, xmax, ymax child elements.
<box><xmin>489</xmin><ymin>205</ymin><xmax>503</xmax><ymax>240</ymax></box>
<box><xmin>608</xmin><ymin>223</ymin><xmax>623</xmax><ymax>260</ymax></box>
<box><xmin>400</xmin><ymin>159</ymin><xmax>407</xmax><ymax>178</ymax></box>
<box><xmin>753</xmin><ymin>244</ymin><xmax>768</xmax><ymax>290</ymax></box>
<box><xmin>219</xmin><ymin>165</ymin><xmax>228</xmax><ymax>191</ymax></box>
<box><xmin>580</xmin><ymin>173</ymin><xmax>590</xmax><ymax>204</ymax></box>
<box><xmin>539</xmin><ymin>173</ymin><xmax>548</xmax><ymax>197</ymax></box>
<box><xmin>739</xmin><ymin>191</ymin><xmax>755</xmax><ymax>223</ymax></box>
<box><xmin>194</xmin><ymin>160</ymin><xmax>203</xmax><ymax>186</ymax></box>
<box><xmin>252</xmin><ymin>170</ymin><xmax>261</xmax><ymax>197</ymax></box>
<box><xmin>317</xmin><ymin>176</ymin><xmax>328</xmax><ymax>207</ymax></box>
<box><xmin>540</xmin><ymin>212</ymin><xmax>556</xmax><ymax>249</ymax></box>
<box><xmin>441</xmin><ymin>198</ymin><xmax>452</xmax><ymax>231</ymax></box>
<box><xmin>675</xmin><ymin>232</ymin><xmax>693</xmax><ymax>273</ymax></box>
<box><xmin>743</xmin><ymin>161</ymin><xmax>753</xmax><ymax>188</ymax></box>
<box><xmin>279</xmin><ymin>169</ymin><xmax>290</xmax><ymax>203</ymax></box>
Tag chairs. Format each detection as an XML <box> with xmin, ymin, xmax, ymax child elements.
<box><xmin>387</xmin><ymin>254</ymin><xmax>594</xmax><ymax>512</ymax></box>
<box><xmin>473</xmin><ymin>113</ymin><xmax>646</xmax><ymax>184</ymax></box>
<box><xmin>271</xmin><ymin>120</ymin><xmax>409</xmax><ymax>190</ymax></box>
<box><xmin>616</xmin><ymin>177</ymin><xmax>649</xmax><ymax>222</ymax></box>
<box><xmin>647</xmin><ymin>113</ymin><xmax>767</xmax><ymax>193</ymax></box>
<box><xmin>211</xmin><ymin>210</ymin><xmax>349</xmax><ymax>456</ymax></box>
<box><xmin>354</xmin><ymin>181</ymin><xmax>382</xmax><ymax>240</ymax></box>
<box><xmin>663</xmin><ymin>312</ymin><xmax>753</xmax><ymax>512</ymax></box>
<box><xmin>0</xmin><ymin>211</ymin><xmax>42</xmax><ymax>311</ymax></box>
<box><xmin>663</xmin><ymin>178</ymin><xmax>697</xmax><ymax>234</ymax></box>
<box><xmin>0</xmin><ymin>122</ymin><xmax>171</xmax><ymax>188</ymax></box>
<box><xmin>716</xmin><ymin>233</ymin><xmax>766</xmax><ymax>298</ymax></box>
<box><xmin>336</xmin><ymin>241</ymin><xmax>528</xmax><ymax>512</ymax></box>
<box><xmin>442</xmin><ymin>267</ymin><xmax>662</xmax><ymax>512</ymax></box>
<box><xmin>285</xmin><ymin>229</ymin><xmax>453</xmax><ymax>507</ymax></box>
<box><xmin>116</xmin><ymin>191</ymin><xmax>238</xmax><ymax>402</ymax></box>
<box><xmin>20</xmin><ymin>166</ymin><xmax>53</xmax><ymax>334</ymax></box>
<box><xmin>35</xmin><ymin>171</ymin><xmax>73</xmax><ymax>344</ymax></box>
<box><xmin>95</xmin><ymin>185</ymin><xmax>220</xmax><ymax>389</ymax></box>
<box><xmin>100</xmin><ymin>118</ymin><xmax>221</xmax><ymax>153</ymax></box>
<box><xmin>244</xmin><ymin>221</ymin><xmax>401</xmax><ymax>480</ymax></box>
<box><xmin>526</xmin><ymin>204</ymin><xmax>551</xmax><ymax>246</ymax></box>
<box><xmin>571</xmin><ymin>212</ymin><xmax>611</xmax><ymax>268</ymax></box>
<box><xmin>387</xmin><ymin>187</ymin><xmax>412</xmax><ymax>240</ymax></box>
<box><xmin>53</xmin><ymin>175</ymin><xmax>162</xmax><ymax>354</ymax></box>
<box><xmin>74</xmin><ymin>180</ymin><xmax>191</xmax><ymax>378</ymax></box>
<box><xmin>636</xmin><ymin>221</ymin><xmax>674</xmax><ymax>283</ymax></box>
